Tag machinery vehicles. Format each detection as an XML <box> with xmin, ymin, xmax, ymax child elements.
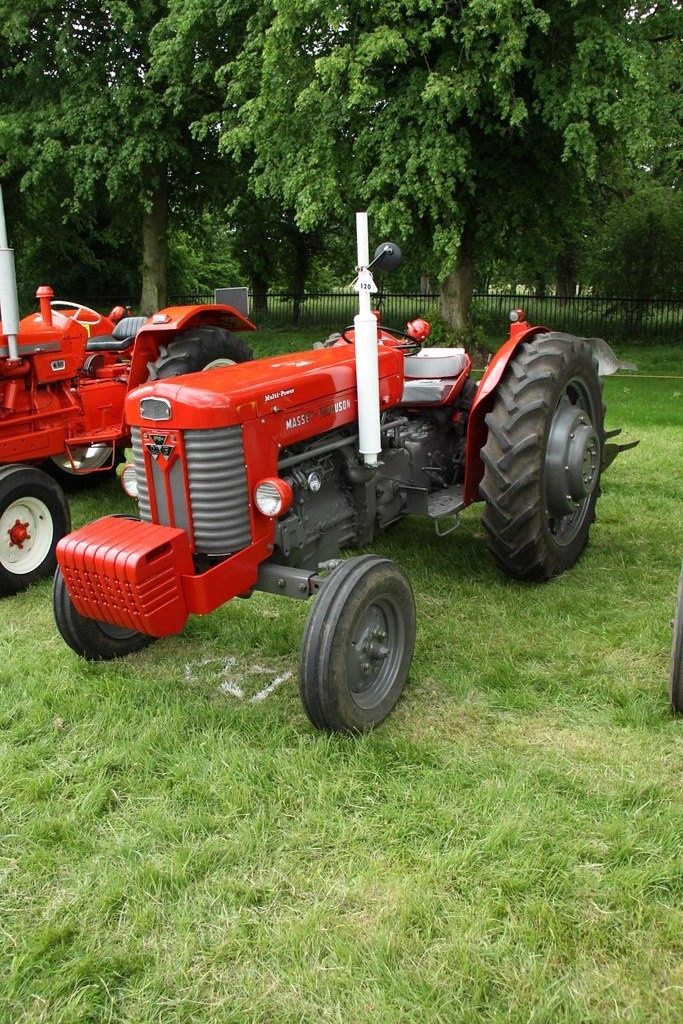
<box><xmin>53</xmin><ymin>213</ymin><xmax>640</xmax><ymax>732</ymax></box>
<box><xmin>0</xmin><ymin>185</ymin><xmax>258</xmax><ymax>596</ymax></box>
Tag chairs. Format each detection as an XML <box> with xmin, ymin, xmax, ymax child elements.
<box><xmin>86</xmin><ymin>314</ymin><xmax>147</xmax><ymax>350</ymax></box>
<box><xmin>400</xmin><ymin>347</ymin><xmax>468</xmax><ymax>402</ymax></box>
